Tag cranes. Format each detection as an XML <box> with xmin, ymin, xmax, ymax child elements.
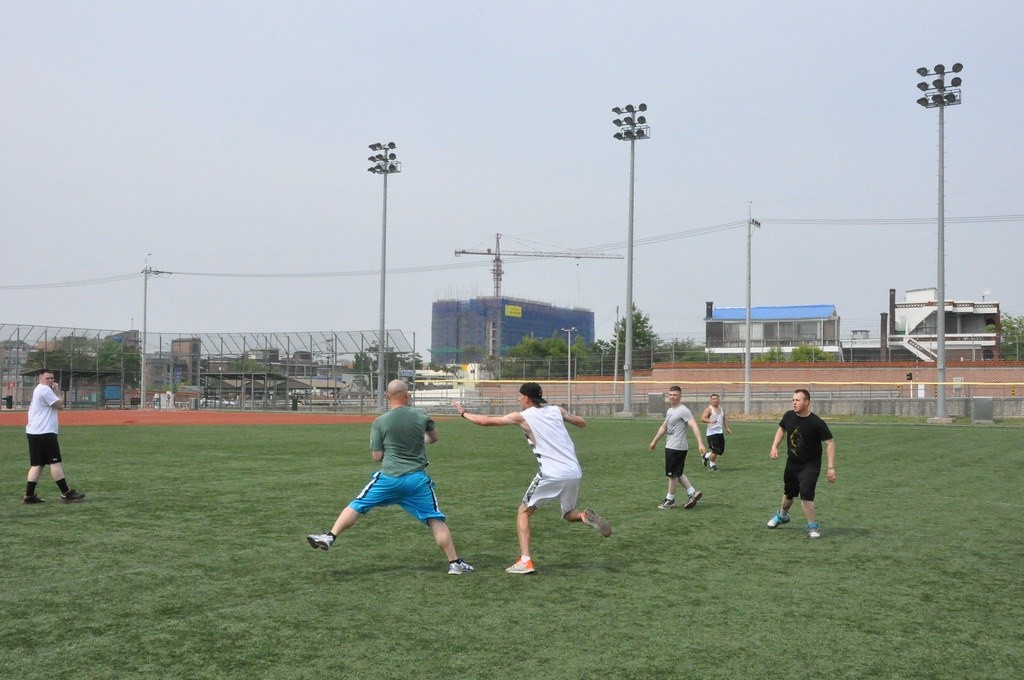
<box><xmin>455</xmin><ymin>233</ymin><xmax>625</xmax><ymax>299</ymax></box>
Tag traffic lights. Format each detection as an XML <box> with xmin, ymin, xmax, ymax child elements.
<box><xmin>907</xmin><ymin>373</ymin><xmax>912</xmax><ymax>380</ymax></box>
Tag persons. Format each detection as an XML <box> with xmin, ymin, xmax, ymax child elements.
<box><xmin>307</xmin><ymin>380</ymin><xmax>474</xmax><ymax>574</ymax></box>
<box><xmin>22</xmin><ymin>369</ymin><xmax>86</xmax><ymax>504</ymax></box>
<box><xmin>767</xmin><ymin>389</ymin><xmax>836</xmax><ymax>538</ymax></box>
<box><xmin>452</xmin><ymin>382</ymin><xmax>612</xmax><ymax>573</ymax></box>
<box><xmin>701</xmin><ymin>393</ymin><xmax>732</xmax><ymax>471</ymax></box>
<box><xmin>648</xmin><ymin>386</ymin><xmax>706</xmax><ymax>509</ymax></box>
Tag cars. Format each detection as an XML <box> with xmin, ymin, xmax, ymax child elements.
<box><xmin>290</xmin><ymin>390</ymin><xmax>305</xmax><ymax>400</ymax></box>
<box><xmin>206</xmin><ymin>396</ymin><xmax>232</xmax><ymax>406</ymax></box>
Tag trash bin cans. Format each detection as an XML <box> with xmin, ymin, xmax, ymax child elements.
<box><xmin>292</xmin><ymin>399</ymin><xmax>297</xmax><ymax>411</ymax></box>
<box><xmin>8</xmin><ymin>396</ymin><xmax>12</xmax><ymax>409</ymax></box>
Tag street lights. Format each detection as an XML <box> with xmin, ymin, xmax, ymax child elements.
<box><xmin>917</xmin><ymin>62</ymin><xmax>963</xmax><ymax>425</ymax></box>
<box><xmin>561</xmin><ymin>327</ymin><xmax>578</xmax><ymax>413</ymax></box>
<box><xmin>612</xmin><ymin>103</ymin><xmax>650</xmax><ymax>420</ymax></box>
<box><xmin>367</xmin><ymin>142</ymin><xmax>402</xmax><ymax>412</ymax></box>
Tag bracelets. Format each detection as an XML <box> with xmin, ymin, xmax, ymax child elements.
<box><xmin>461</xmin><ymin>411</ymin><xmax>468</xmax><ymax>417</ymax></box>
<box><xmin>828</xmin><ymin>468</ymin><xmax>834</xmax><ymax>469</ymax></box>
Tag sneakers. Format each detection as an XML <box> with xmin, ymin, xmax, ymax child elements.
<box><xmin>448</xmin><ymin>559</ymin><xmax>474</xmax><ymax>575</ymax></box>
<box><xmin>806</xmin><ymin>522</ymin><xmax>822</xmax><ymax>539</ymax></box>
<box><xmin>21</xmin><ymin>493</ymin><xmax>45</xmax><ymax>505</ymax></box>
<box><xmin>307</xmin><ymin>533</ymin><xmax>334</xmax><ymax>551</ymax></box>
<box><xmin>767</xmin><ymin>511</ymin><xmax>790</xmax><ymax>529</ymax></box>
<box><xmin>708</xmin><ymin>466</ymin><xmax>719</xmax><ymax>473</ymax></box>
<box><xmin>684</xmin><ymin>491</ymin><xmax>702</xmax><ymax>509</ymax></box>
<box><xmin>506</xmin><ymin>557</ymin><xmax>536</xmax><ymax>574</ymax></box>
<box><xmin>580</xmin><ymin>509</ymin><xmax>612</xmax><ymax>538</ymax></box>
<box><xmin>701</xmin><ymin>452</ymin><xmax>709</xmax><ymax>467</ymax></box>
<box><xmin>61</xmin><ymin>488</ymin><xmax>85</xmax><ymax>504</ymax></box>
<box><xmin>658</xmin><ymin>498</ymin><xmax>676</xmax><ymax>510</ymax></box>
